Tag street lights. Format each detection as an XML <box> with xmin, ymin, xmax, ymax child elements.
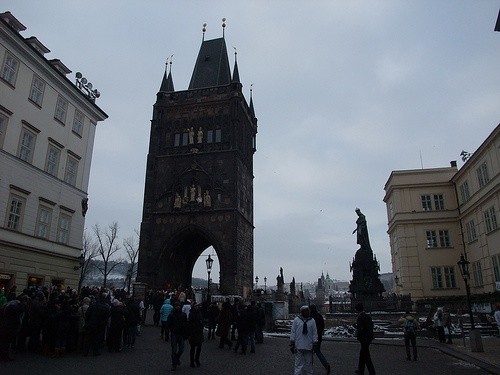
<box><xmin>456</xmin><ymin>254</ymin><xmax>484</xmax><ymax>352</ymax></box>
<box><xmin>206</xmin><ymin>254</ymin><xmax>214</xmax><ymax>305</ymax></box>
<box><xmin>264</xmin><ymin>277</ymin><xmax>267</xmax><ymax>293</ymax></box>
<box><xmin>255</xmin><ymin>276</ymin><xmax>259</xmax><ymax>289</ymax></box>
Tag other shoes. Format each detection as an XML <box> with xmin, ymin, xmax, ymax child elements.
<box><xmin>172</xmin><ymin>363</ymin><xmax>180</xmax><ymax>370</ymax></box>
<box><xmin>233</xmin><ymin>347</ymin><xmax>238</xmax><ymax>353</ymax></box>
<box><xmin>190</xmin><ymin>361</ymin><xmax>201</xmax><ymax>367</ymax></box>
<box><xmin>219</xmin><ymin>343</ymin><xmax>224</xmax><ymax>348</ymax></box>
<box><xmin>354</xmin><ymin>369</ymin><xmax>364</xmax><ymax>375</ymax></box>
<box><xmin>240</xmin><ymin>350</ymin><xmax>246</xmax><ymax>355</ymax></box>
<box><xmin>228</xmin><ymin>341</ymin><xmax>233</xmax><ymax>350</ymax></box>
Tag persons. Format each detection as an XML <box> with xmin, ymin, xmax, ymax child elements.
<box><xmin>352</xmin><ymin>208</ymin><xmax>371</xmax><ymax>249</ymax></box>
<box><xmin>494</xmin><ymin>303</ymin><xmax>500</xmax><ymax>338</ymax></box>
<box><xmin>434</xmin><ymin>307</ymin><xmax>454</xmax><ymax>345</ymax></box>
<box><xmin>309</xmin><ymin>305</ymin><xmax>331</xmax><ymax>375</ymax></box>
<box><xmin>354</xmin><ymin>305</ymin><xmax>377</xmax><ymax>375</ymax></box>
<box><xmin>399</xmin><ymin>308</ymin><xmax>421</xmax><ymax>362</ymax></box>
<box><xmin>0</xmin><ymin>276</ymin><xmax>265</xmax><ymax>371</ymax></box>
<box><xmin>289</xmin><ymin>305</ymin><xmax>318</xmax><ymax>375</ymax></box>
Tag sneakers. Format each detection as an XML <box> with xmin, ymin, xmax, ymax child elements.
<box><xmin>326</xmin><ymin>363</ymin><xmax>330</xmax><ymax>375</ymax></box>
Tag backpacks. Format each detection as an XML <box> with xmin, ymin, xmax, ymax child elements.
<box><xmin>405</xmin><ymin>317</ymin><xmax>417</xmax><ymax>336</ymax></box>
